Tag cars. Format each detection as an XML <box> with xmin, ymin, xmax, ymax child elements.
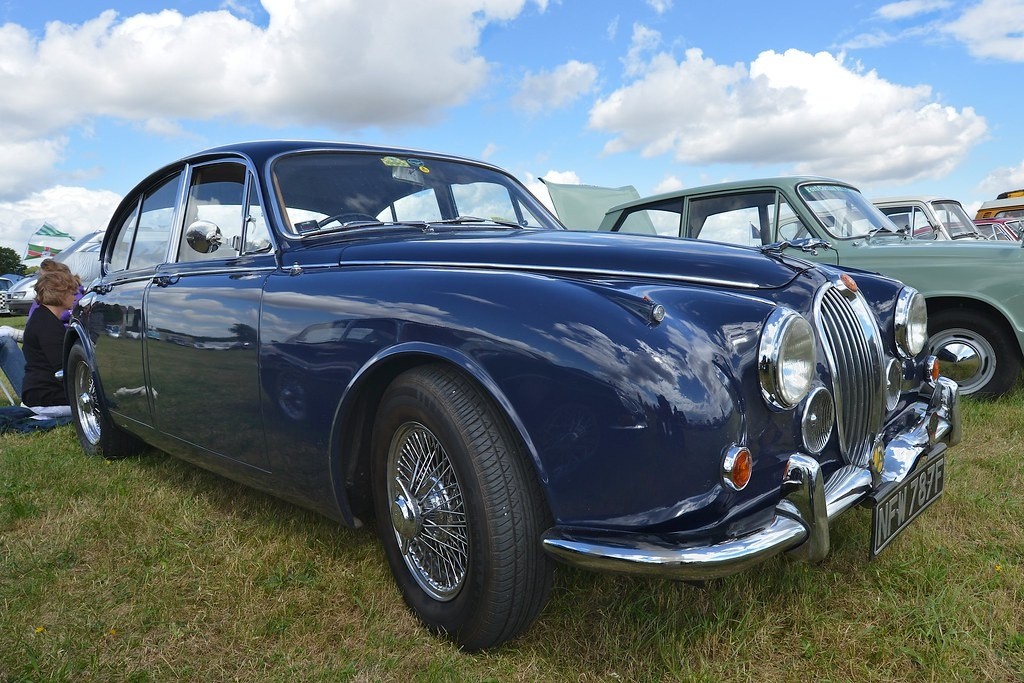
<box><xmin>0</xmin><ymin>277</ymin><xmax>14</xmax><ymax>314</ymax></box>
<box><xmin>974</xmin><ymin>189</ymin><xmax>1024</xmax><ymax>219</ymax></box>
<box><xmin>532</xmin><ymin>175</ymin><xmax>1024</xmax><ymax>404</ymax></box>
<box><xmin>62</xmin><ymin>137</ymin><xmax>963</xmax><ymax>660</ymax></box>
<box><xmin>912</xmin><ymin>218</ymin><xmax>1024</xmax><ymax>242</ymax></box>
<box><xmin>768</xmin><ymin>196</ymin><xmax>989</xmax><ymax>241</ymax></box>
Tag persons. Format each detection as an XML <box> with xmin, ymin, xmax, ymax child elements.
<box><xmin>20</xmin><ymin>259</ymin><xmax>86</xmax><ymax>418</ymax></box>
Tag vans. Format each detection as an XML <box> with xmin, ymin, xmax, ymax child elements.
<box><xmin>4</xmin><ymin>229</ymin><xmax>237</xmax><ymax>316</ymax></box>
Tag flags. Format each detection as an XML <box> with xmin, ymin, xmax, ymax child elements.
<box><xmin>33</xmin><ymin>222</ymin><xmax>75</xmax><ymax>241</ymax></box>
<box><xmin>24</xmin><ymin>244</ymin><xmax>61</xmax><ymax>260</ymax></box>
